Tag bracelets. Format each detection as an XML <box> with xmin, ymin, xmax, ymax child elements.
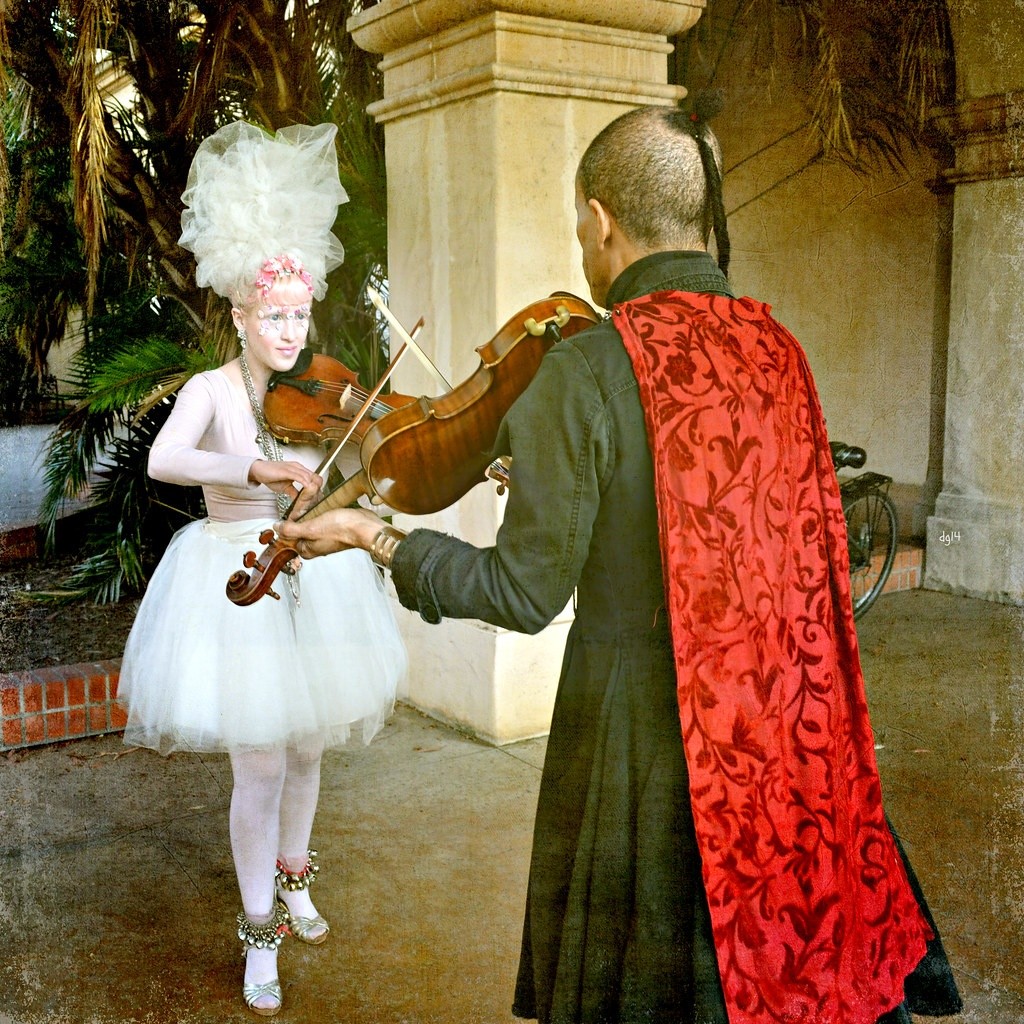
<box><xmin>369</xmin><ymin>525</ymin><xmax>409</xmax><ymax>571</ymax></box>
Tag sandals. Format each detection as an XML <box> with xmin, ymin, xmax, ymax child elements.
<box><xmin>275</xmin><ymin>887</ymin><xmax>330</xmax><ymax>946</ymax></box>
<box><xmin>243</xmin><ymin>940</ymin><xmax>284</xmax><ymax>1017</ymax></box>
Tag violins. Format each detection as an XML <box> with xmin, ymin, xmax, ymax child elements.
<box><xmin>224</xmin><ymin>289</ymin><xmax>601</xmax><ymax>608</ymax></box>
<box><xmin>260</xmin><ymin>350</ymin><xmax>513</xmax><ymax>496</ymax></box>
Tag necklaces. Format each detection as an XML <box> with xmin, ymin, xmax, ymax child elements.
<box><xmin>240</xmin><ymin>347</ymin><xmax>301</xmax><ymax>611</ymax></box>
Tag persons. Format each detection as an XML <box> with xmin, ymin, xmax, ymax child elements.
<box><xmin>273</xmin><ymin>106</ymin><xmax>968</xmax><ymax>1024</ymax></box>
<box><xmin>115</xmin><ymin>117</ymin><xmax>407</xmax><ymax>1017</ymax></box>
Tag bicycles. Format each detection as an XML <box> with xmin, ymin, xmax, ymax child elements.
<box><xmin>828</xmin><ymin>442</ymin><xmax>898</xmax><ymax>623</ymax></box>
